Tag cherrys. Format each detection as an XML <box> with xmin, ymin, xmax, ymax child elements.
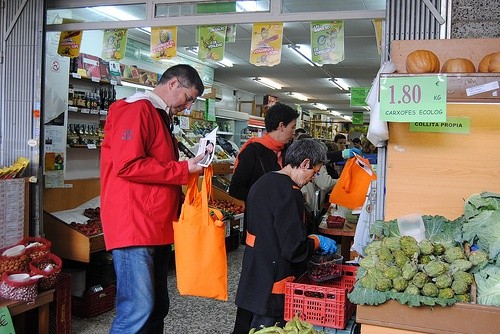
<box><xmin>70</xmin><ymin>207</ymin><xmax>103</xmax><ymax>236</ymax></box>
<box><xmin>326</xmin><ymin>215</ymin><xmax>345</xmax><ymax>228</ymax></box>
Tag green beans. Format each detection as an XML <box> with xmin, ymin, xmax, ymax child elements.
<box><xmin>248</xmin><ymin>313</ymin><xmax>328</xmax><ymax>334</ymax></box>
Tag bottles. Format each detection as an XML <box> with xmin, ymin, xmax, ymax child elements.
<box><xmin>67</xmin><ymin>123</ymin><xmax>97</xmax><ymax>146</ymax></box>
<box><xmin>69</xmin><ymin>83</ymin><xmax>117</xmax><ymax>111</ymax></box>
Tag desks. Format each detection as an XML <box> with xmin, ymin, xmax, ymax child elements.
<box><xmin>0</xmin><ymin>288</ymin><xmax>55</xmax><ymax>334</ymax></box>
<box><xmin>357</xmin><ymin>299</ymin><xmax>500</xmax><ymax>334</ymax></box>
<box><xmin>318</xmin><ymin>204</ymin><xmax>356</xmax><ymax>265</ymax></box>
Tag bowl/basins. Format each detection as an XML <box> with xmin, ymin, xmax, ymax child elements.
<box><xmin>307</xmin><ymin>253</ymin><xmax>344</xmax><ymax>282</ymax></box>
<box><xmin>326</xmin><ymin>219</ymin><xmax>345</xmax><ymax>229</ymax></box>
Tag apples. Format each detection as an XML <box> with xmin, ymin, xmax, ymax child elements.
<box><xmin>210</xmin><ymin>199</ymin><xmax>243</xmax><ymax>214</ymax></box>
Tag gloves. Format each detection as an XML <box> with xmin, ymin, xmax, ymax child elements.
<box><xmin>314</xmin><ymin>235</ymin><xmax>338</xmax><ymax>256</ymax></box>
<box><xmin>342</xmin><ymin>148</ymin><xmax>362</xmax><ymax>159</ymax></box>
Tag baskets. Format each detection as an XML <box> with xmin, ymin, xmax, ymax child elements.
<box><xmin>71</xmin><ymin>284</ymin><xmax>115</xmax><ymax>317</ymax></box>
<box><xmin>284</xmin><ymin>265</ymin><xmax>360</xmax><ymax>330</ymax></box>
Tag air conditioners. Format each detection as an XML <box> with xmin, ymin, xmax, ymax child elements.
<box><xmin>214</xmin><ymin>85</ymin><xmax>223</xmax><ymax>102</ymax></box>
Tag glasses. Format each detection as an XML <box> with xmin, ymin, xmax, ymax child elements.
<box><xmin>176</xmin><ymin>77</ymin><xmax>196</xmax><ymax>104</ymax></box>
<box><xmin>313</xmin><ymin>166</ymin><xmax>320</xmax><ymax>178</ymax></box>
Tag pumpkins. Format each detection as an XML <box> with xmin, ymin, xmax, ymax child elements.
<box><xmin>405</xmin><ymin>50</ymin><xmax>440</xmax><ymax>73</ymax></box>
<box><xmin>478</xmin><ymin>52</ymin><xmax>500</xmax><ymax>73</ymax></box>
<box><xmin>441</xmin><ymin>58</ymin><xmax>476</xmax><ymax>73</ymax></box>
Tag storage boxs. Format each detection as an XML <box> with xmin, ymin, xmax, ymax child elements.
<box><xmin>72</xmin><ymin>261</ymin><xmax>115</xmax><ymax>318</ymax></box>
<box><xmin>284</xmin><ymin>264</ymin><xmax>357</xmax><ymax>329</ymax></box>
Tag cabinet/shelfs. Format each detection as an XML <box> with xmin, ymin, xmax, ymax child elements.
<box><xmin>68</xmin><ymin>73</ymin><xmax>339</xmax><ymax>147</ymax></box>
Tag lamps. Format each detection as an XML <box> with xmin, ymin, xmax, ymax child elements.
<box><xmin>253</xmin><ymin>77</ymin><xmax>282</xmax><ymax>90</ymax></box>
<box><xmin>88</xmin><ymin>6</ymin><xmax>150</xmax><ymax>35</ymax></box>
<box><xmin>287</xmin><ymin>44</ymin><xmax>370</xmax><ymax>112</ymax></box>
<box><xmin>186</xmin><ymin>45</ymin><xmax>233</xmax><ymax>68</ymax></box>
<box><xmin>312</xmin><ymin>103</ymin><xmax>328</xmax><ymax>111</ymax></box>
<box><xmin>287</xmin><ymin>91</ymin><xmax>308</xmax><ymax>102</ymax></box>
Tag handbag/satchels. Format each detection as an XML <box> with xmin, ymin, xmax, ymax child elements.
<box><xmin>329</xmin><ymin>151</ymin><xmax>377</xmax><ymax>210</ymax></box>
<box><xmin>173</xmin><ymin>163</ymin><xmax>228</xmax><ymax>301</ymax></box>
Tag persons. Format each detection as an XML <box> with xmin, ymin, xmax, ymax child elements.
<box><xmin>228</xmin><ymin>101</ymin><xmax>300</xmax><ymax>246</ymax></box>
<box><xmin>99</xmin><ymin>64</ymin><xmax>206</xmax><ymax>334</ymax></box>
<box><xmin>233</xmin><ymin>138</ymin><xmax>338</xmax><ymax>334</ymax></box>
<box><xmin>282</xmin><ymin>128</ymin><xmax>377</xmax><ymax>238</ymax></box>
<box><xmin>199</xmin><ymin>142</ymin><xmax>214</xmax><ymax>164</ymax></box>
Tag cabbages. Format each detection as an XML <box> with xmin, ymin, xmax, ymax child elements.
<box><xmin>347</xmin><ymin>214</ymin><xmax>468</xmax><ymax>306</ymax></box>
<box><xmin>460</xmin><ymin>191</ymin><xmax>500</xmax><ymax>307</ymax></box>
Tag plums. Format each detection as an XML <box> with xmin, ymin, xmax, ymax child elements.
<box><xmin>304</xmin><ymin>260</ymin><xmax>341</xmax><ymax>299</ymax></box>
